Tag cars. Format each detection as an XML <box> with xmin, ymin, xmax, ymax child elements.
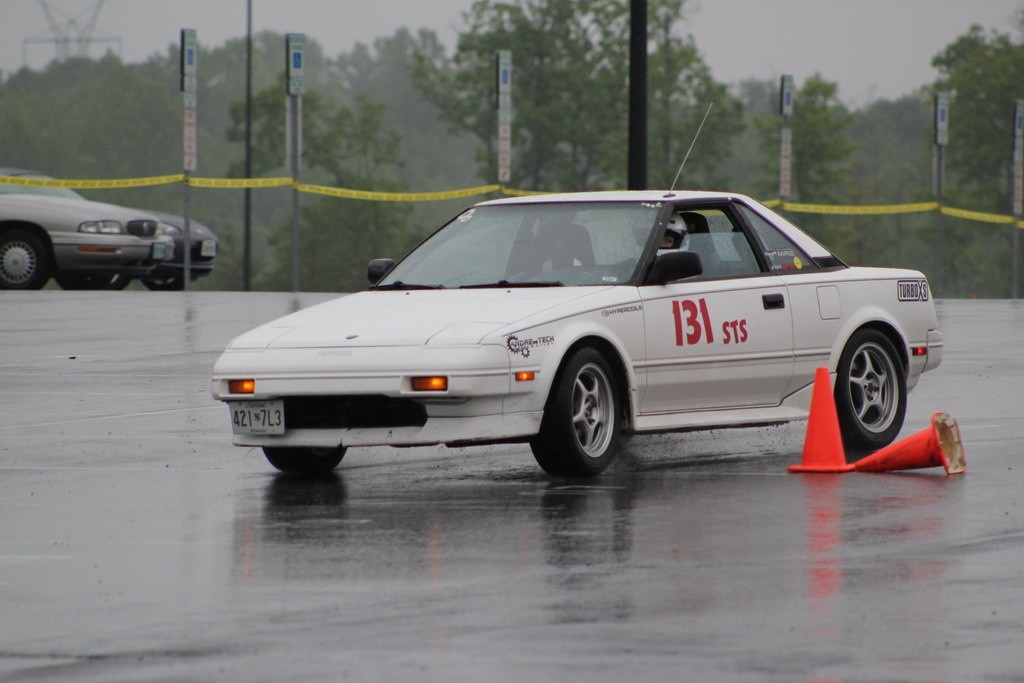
<box><xmin>210</xmin><ymin>188</ymin><xmax>942</xmax><ymax>481</ymax></box>
<box><xmin>0</xmin><ymin>168</ymin><xmax>175</xmax><ymax>289</ymax></box>
<box><xmin>88</xmin><ymin>207</ymin><xmax>222</xmax><ymax>291</ymax></box>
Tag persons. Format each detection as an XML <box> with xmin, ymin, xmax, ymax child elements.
<box><xmin>620</xmin><ymin>214</ymin><xmax>697</xmax><ymax>281</ymax></box>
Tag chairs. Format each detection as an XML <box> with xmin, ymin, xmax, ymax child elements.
<box><xmin>674</xmin><ymin>211</ymin><xmax>715</xmax><ymax>280</ymax></box>
<box><xmin>529</xmin><ymin>220</ymin><xmax>594</xmax><ymax>275</ymax></box>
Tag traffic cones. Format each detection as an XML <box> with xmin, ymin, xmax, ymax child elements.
<box><xmin>854</xmin><ymin>412</ymin><xmax>970</xmax><ymax>474</ymax></box>
<box><xmin>787</xmin><ymin>367</ymin><xmax>857</xmax><ymax>474</ymax></box>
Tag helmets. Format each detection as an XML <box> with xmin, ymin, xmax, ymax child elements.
<box><xmin>630</xmin><ymin>207</ymin><xmax>689</xmax><ymax>259</ymax></box>
